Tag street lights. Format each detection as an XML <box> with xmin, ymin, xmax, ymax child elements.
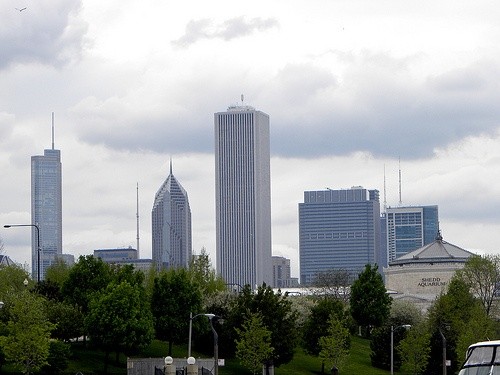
<box><xmin>390</xmin><ymin>324</ymin><xmax>411</xmax><ymax>375</ymax></box>
<box><xmin>3</xmin><ymin>224</ymin><xmax>40</xmax><ymax>282</ymax></box>
<box><xmin>187</xmin><ymin>312</ymin><xmax>215</xmax><ymax>358</ymax></box>
